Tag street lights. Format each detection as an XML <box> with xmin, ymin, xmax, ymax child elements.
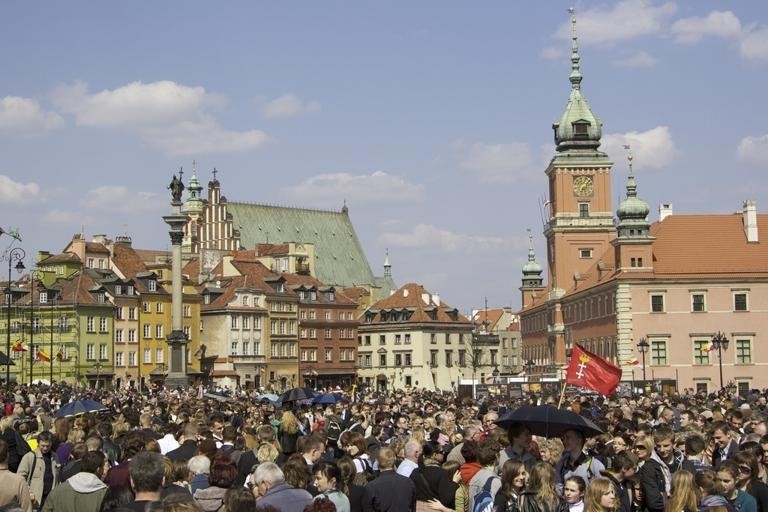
<box><xmin>492</xmin><ymin>362</ymin><xmax>500</xmax><ymax>382</ymax></box>
<box><xmin>50</xmin><ymin>288</ymin><xmax>64</xmax><ymax>387</ymax></box>
<box><xmin>524</xmin><ymin>359</ymin><xmax>536</xmax><ymax>381</ymax></box>
<box><xmin>28</xmin><ymin>268</ymin><xmax>47</xmax><ymax>388</ymax></box>
<box><xmin>636</xmin><ymin>336</ymin><xmax>651</xmax><ymax>388</ymax></box>
<box><xmin>711</xmin><ymin>329</ymin><xmax>730</xmax><ymax>389</ymax></box>
<box><xmin>6</xmin><ymin>245</ymin><xmax>27</xmax><ymax>395</ymax></box>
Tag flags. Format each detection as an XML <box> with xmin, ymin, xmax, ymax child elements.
<box><xmin>36</xmin><ymin>351</ymin><xmax>51</xmax><ymax>362</ymax></box>
<box><xmin>566</xmin><ymin>342</ymin><xmax>623</xmax><ymax>396</ymax></box>
<box><xmin>12</xmin><ymin>340</ymin><xmax>28</xmax><ymax>352</ymax></box>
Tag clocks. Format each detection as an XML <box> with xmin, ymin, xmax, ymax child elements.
<box><xmin>572</xmin><ymin>173</ymin><xmax>595</xmax><ymax>198</ymax></box>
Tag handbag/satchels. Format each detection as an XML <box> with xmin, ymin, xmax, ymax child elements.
<box><xmin>473</xmin><ymin>476</ymin><xmax>494</xmax><ymax>512</ymax></box>
<box><xmin>323</xmin><ymin>416</ymin><xmax>340</xmax><ymax>440</ymax></box>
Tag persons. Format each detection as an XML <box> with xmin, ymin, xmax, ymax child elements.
<box><xmin>1</xmin><ymin>375</ymin><xmax>768</xmax><ymax>511</ymax></box>
<box><xmin>166</xmin><ymin>174</ymin><xmax>185</xmax><ymax>202</ymax></box>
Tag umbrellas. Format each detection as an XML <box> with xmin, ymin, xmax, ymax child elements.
<box><xmin>0</xmin><ymin>350</ymin><xmax>16</xmax><ymax>365</ymax></box>
